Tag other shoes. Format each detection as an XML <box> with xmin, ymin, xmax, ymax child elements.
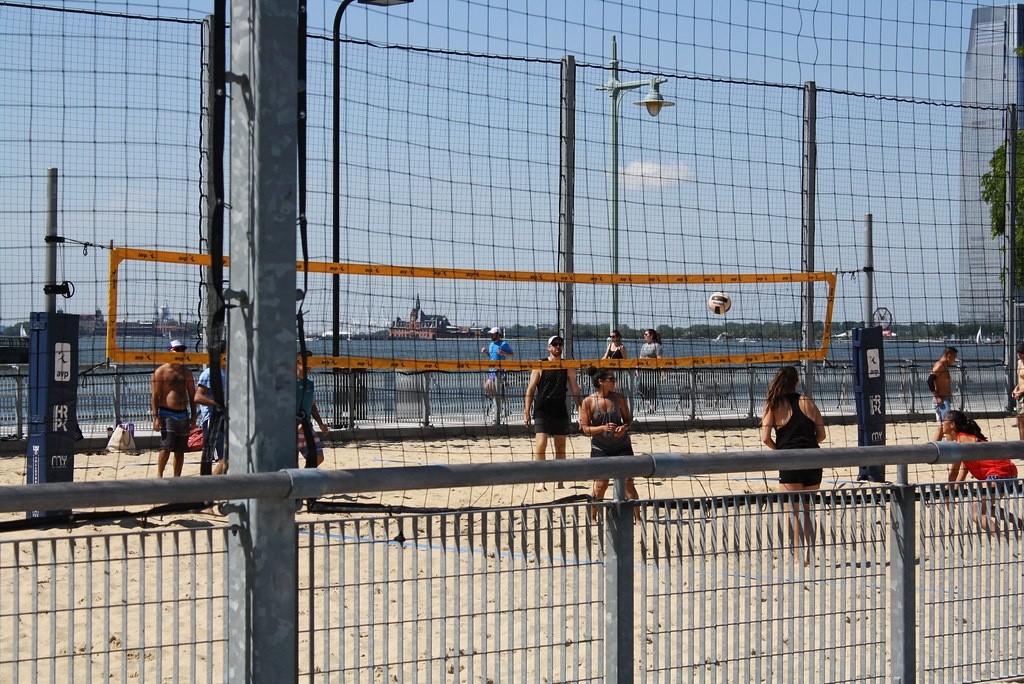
<box><xmin>502</xmin><ymin>411</ymin><xmax>512</xmax><ymax>418</ymax></box>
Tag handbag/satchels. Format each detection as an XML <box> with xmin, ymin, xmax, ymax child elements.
<box><xmin>106</xmin><ymin>424</ymin><xmax>136</xmax><ymax>451</ymax></box>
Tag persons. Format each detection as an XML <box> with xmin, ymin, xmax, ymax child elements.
<box><xmin>296</xmin><ymin>349</ymin><xmax>329</xmax><ymax>470</ymax></box>
<box><xmin>579</xmin><ymin>363</ymin><xmax>643</xmax><ymax>527</ymax></box>
<box><xmin>152</xmin><ymin>337</ymin><xmax>196</xmax><ymax>479</ymax></box>
<box><xmin>193</xmin><ymin>340</ymin><xmax>225</xmax><ymax>475</ymax></box>
<box><xmin>523</xmin><ymin>334</ymin><xmax>583</xmax><ymax>494</ymax></box>
<box><xmin>927</xmin><ymin>346</ymin><xmax>958</xmax><ymax>440</ymax></box>
<box><xmin>601</xmin><ymin>328</ymin><xmax>666</xmax><ymax>413</ymax></box>
<box><xmin>761</xmin><ymin>366</ymin><xmax>827</xmax><ymax>569</ymax></box>
<box><xmin>941</xmin><ymin>410</ymin><xmax>1024</xmax><ymax>544</ymax></box>
<box><xmin>1011</xmin><ymin>342</ymin><xmax>1024</xmax><ymax>442</ymax></box>
<box><xmin>481</xmin><ymin>327</ymin><xmax>515</xmax><ymax>418</ymax></box>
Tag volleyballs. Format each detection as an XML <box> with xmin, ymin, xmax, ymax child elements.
<box><xmin>708</xmin><ymin>291</ymin><xmax>732</xmax><ymax>315</ymax></box>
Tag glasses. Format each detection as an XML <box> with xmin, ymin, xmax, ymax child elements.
<box><xmin>609</xmin><ymin>336</ymin><xmax>615</xmax><ymax>338</ymax></box>
<box><xmin>173</xmin><ymin>347</ymin><xmax>185</xmax><ymax>352</ymax></box>
<box><xmin>552</xmin><ymin>342</ymin><xmax>562</xmax><ymax>347</ymax></box>
<box><xmin>602</xmin><ymin>377</ymin><xmax>617</xmax><ymax>383</ymax></box>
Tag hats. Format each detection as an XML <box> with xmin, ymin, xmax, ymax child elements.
<box><xmin>1015</xmin><ymin>346</ymin><xmax>1024</xmax><ymax>355</ymax></box>
<box><xmin>167</xmin><ymin>339</ymin><xmax>187</xmax><ymax>350</ymax></box>
<box><xmin>488</xmin><ymin>327</ymin><xmax>501</xmax><ymax>334</ymax></box>
<box><xmin>548</xmin><ymin>336</ymin><xmax>563</xmax><ymax>345</ymax></box>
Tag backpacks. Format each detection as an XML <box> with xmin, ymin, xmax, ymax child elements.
<box><xmin>187</xmin><ymin>428</ymin><xmax>204</xmax><ymax>451</ymax></box>
